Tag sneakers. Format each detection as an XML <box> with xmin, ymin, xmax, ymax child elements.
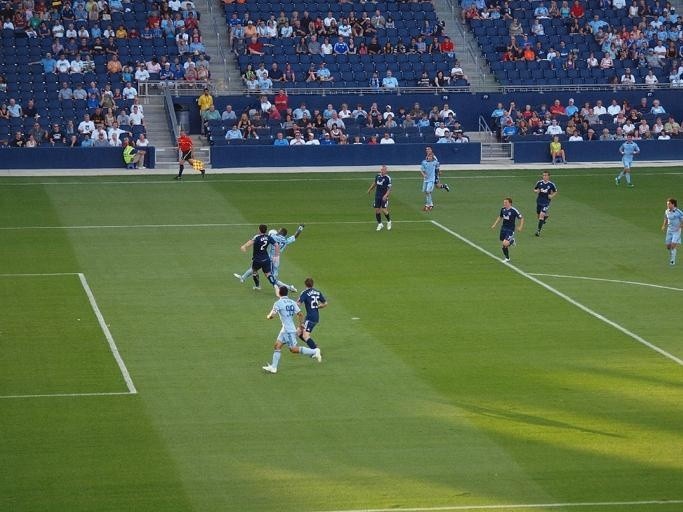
<box><xmin>387</xmin><ymin>220</ymin><xmax>391</xmax><ymax>230</ymax></box>
<box><xmin>444</xmin><ymin>184</ymin><xmax>450</xmax><ymax>191</ymax></box>
<box><xmin>512</xmin><ymin>238</ymin><xmax>515</xmax><ymax>245</ymax></box>
<box><xmin>234</xmin><ymin>274</ymin><xmax>244</xmax><ymax>282</ymax></box>
<box><xmin>311</xmin><ymin>344</ymin><xmax>321</xmax><ymax>362</ymax></box>
<box><xmin>263</xmin><ymin>366</ymin><xmax>277</xmax><ymax>373</ymax></box>
<box><xmin>503</xmin><ymin>258</ymin><xmax>510</xmax><ymax>262</ymax></box>
<box><xmin>536</xmin><ymin>230</ymin><xmax>540</xmax><ymax>235</ymax></box>
<box><xmin>290</xmin><ymin>285</ymin><xmax>297</xmax><ymax>292</ymax></box>
<box><xmin>376</xmin><ymin>223</ymin><xmax>383</xmax><ymax>231</ymax></box>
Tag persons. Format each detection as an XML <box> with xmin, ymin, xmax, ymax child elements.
<box><xmin>239</xmin><ymin>224</ymin><xmax>280</xmax><ymax>298</ymax></box>
<box><xmin>532</xmin><ymin>170</ymin><xmax>559</xmax><ymax>237</ymax></box>
<box><xmin>198</xmin><ymin>88</ymin><xmax>468</xmax><ymax>145</ymax></box>
<box><xmin>233</xmin><ymin>222</ymin><xmax>305</xmax><ymax>293</ymax></box>
<box><xmin>261</xmin><ymin>285</ymin><xmax>322</xmax><ymax>374</ymax></box>
<box><xmin>587</xmin><ymin>1</ymin><xmax>683</xmax><ymax>88</ymax></box>
<box><xmin>368</xmin><ymin>165</ymin><xmax>393</xmax><ymax>232</ymax></box>
<box><xmin>123</xmin><ymin>140</ymin><xmax>146</xmax><ymax>168</ymax></box>
<box><xmin>615</xmin><ymin>135</ymin><xmax>640</xmax><ymax>186</ymax></box>
<box><xmin>462</xmin><ymin>0</ymin><xmax>613</xmax><ymax>69</ymax></box>
<box><xmin>550</xmin><ymin>137</ymin><xmax>567</xmax><ymax>164</ymax></box>
<box><xmin>426</xmin><ymin>146</ymin><xmax>449</xmax><ymax>191</ymax></box>
<box><xmin>662</xmin><ymin>198</ymin><xmax>683</xmax><ymax>267</ymax></box>
<box><xmin>420</xmin><ymin>153</ymin><xmax>440</xmax><ymax>211</ymax></box>
<box><xmin>225</xmin><ymin>0</ymin><xmax>463</xmax><ymax>94</ymax></box>
<box><xmin>297</xmin><ymin>278</ymin><xmax>328</xmax><ymax>349</ymax></box>
<box><xmin>492</xmin><ymin>97</ymin><xmax>682</xmax><ymax>142</ymax></box>
<box><xmin>175</xmin><ymin>130</ymin><xmax>205</xmax><ymax>179</ymax></box>
<box><xmin>487</xmin><ymin>197</ymin><xmax>525</xmax><ymax>262</ymax></box>
<box><xmin>1</xmin><ymin>0</ymin><xmax>210</xmax><ymax>147</ymax></box>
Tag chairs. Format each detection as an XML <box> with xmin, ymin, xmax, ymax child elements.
<box><xmin>0</xmin><ymin>0</ymin><xmax>683</xmax><ymax>148</ymax></box>
